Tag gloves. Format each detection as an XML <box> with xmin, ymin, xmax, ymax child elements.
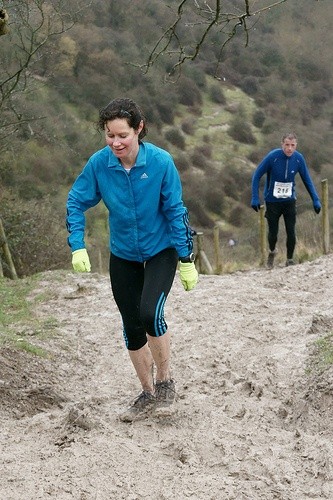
<box><xmin>179</xmin><ymin>260</ymin><xmax>198</xmax><ymax>291</ymax></box>
<box><xmin>313</xmin><ymin>201</ymin><xmax>321</xmax><ymax>214</ymax></box>
<box><xmin>72</xmin><ymin>248</ymin><xmax>91</xmax><ymax>273</ymax></box>
<box><xmin>251</xmin><ymin>200</ymin><xmax>260</xmax><ymax>212</ymax></box>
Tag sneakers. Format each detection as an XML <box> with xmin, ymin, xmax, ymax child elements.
<box><xmin>119</xmin><ymin>391</ymin><xmax>158</xmax><ymax>422</ymax></box>
<box><xmin>155</xmin><ymin>378</ymin><xmax>175</xmax><ymax>406</ymax></box>
<box><xmin>285</xmin><ymin>259</ymin><xmax>296</xmax><ymax>267</ymax></box>
<box><xmin>265</xmin><ymin>252</ymin><xmax>275</xmax><ymax>270</ymax></box>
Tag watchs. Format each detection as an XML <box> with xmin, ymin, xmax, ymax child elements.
<box><xmin>180</xmin><ymin>253</ymin><xmax>196</xmax><ymax>263</ymax></box>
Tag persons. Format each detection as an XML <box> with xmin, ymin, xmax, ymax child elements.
<box><xmin>65</xmin><ymin>94</ymin><xmax>200</xmax><ymax>424</ymax></box>
<box><xmin>250</xmin><ymin>131</ymin><xmax>322</xmax><ymax>270</ymax></box>
